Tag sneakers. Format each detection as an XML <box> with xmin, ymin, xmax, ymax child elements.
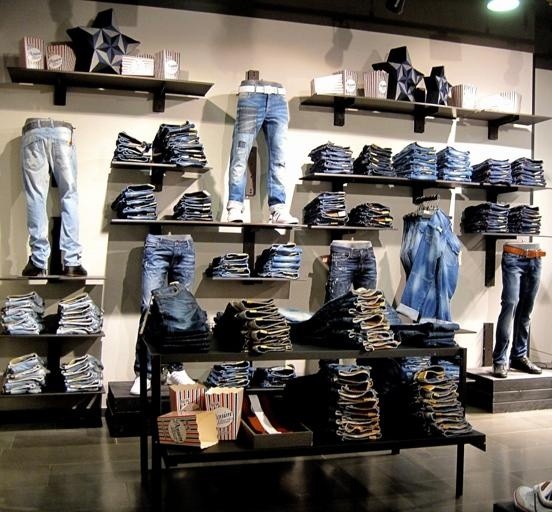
<box><xmin>268</xmin><ymin>203</ymin><xmax>298</xmax><ymax>224</ymax></box>
<box><xmin>513</xmin><ymin>480</ymin><xmax>552</xmax><ymax>512</ymax></box>
<box><xmin>166</xmin><ymin>370</ymin><xmax>195</xmax><ymax>385</ymax></box>
<box><xmin>22</xmin><ymin>255</ymin><xmax>47</xmax><ymax>277</ymax></box>
<box><xmin>130</xmin><ymin>375</ymin><xmax>152</xmax><ymax>396</ymax></box>
<box><xmin>226</xmin><ymin>200</ymin><xmax>245</xmax><ymax>221</ymax></box>
<box><xmin>64</xmin><ymin>265</ymin><xmax>88</xmax><ymax>277</ymax></box>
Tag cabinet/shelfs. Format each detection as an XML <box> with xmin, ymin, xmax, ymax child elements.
<box><xmin>143</xmin><ymin>325</ymin><xmax>487</xmax><ymax>501</ymax></box>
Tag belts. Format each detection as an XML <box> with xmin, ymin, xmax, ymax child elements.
<box><xmin>22</xmin><ymin>121</ymin><xmax>73</xmax><ymax>129</ymax></box>
<box><xmin>503</xmin><ymin>245</ymin><xmax>546</xmax><ymax>257</ymax></box>
<box><xmin>238</xmin><ymin>85</ymin><xmax>287</xmax><ymax>95</ymax></box>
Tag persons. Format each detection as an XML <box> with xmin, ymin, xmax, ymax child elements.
<box><xmin>19</xmin><ymin>116</ymin><xmax>87</xmax><ymax>278</ymax></box>
<box><xmin>325</xmin><ymin>240</ymin><xmax>376</xmax><ymax>305</ymax></box>
<box><xmin>226</xmin><ymin>79</ymin><xmax>301</xmax><ymax>223</ymax></box>
<box><xmin>129</xmin><ymin>234</ymin><xmax>197</xmax><ymax>395</ymax></box>
<box><xmin>493</xmin><ymin>243</ymin><xmax>547</xmax><ymax>377</ymax></box>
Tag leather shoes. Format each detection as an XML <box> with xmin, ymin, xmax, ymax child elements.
<box><xmin>493</xmin><ymin>356</ymin><xmax>543</xmax><ymax>378</ymax></box>
